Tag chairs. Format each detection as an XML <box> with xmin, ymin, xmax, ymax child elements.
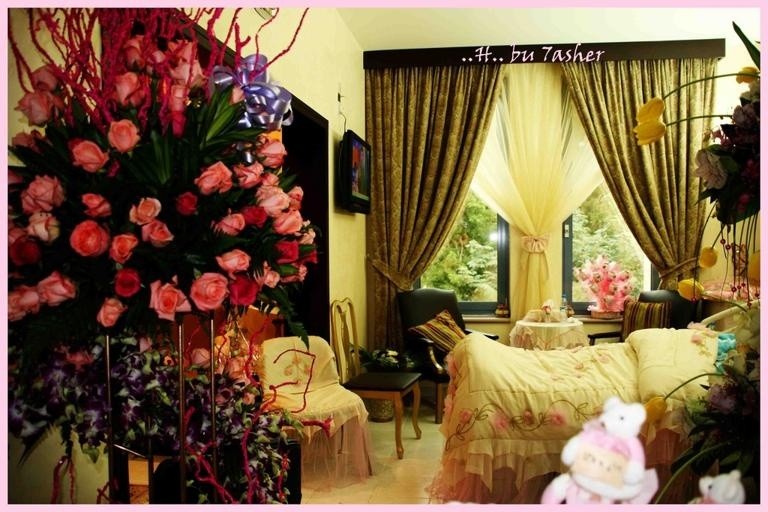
<box><xmin>587</xmin><ymin>289</ymin><xmax>698</xmax><ymax>345</ymax></box>
<box><xmin>255</xmin><ymin>286</ymin><xmax>501</xmax><ymax>486</ymax></box>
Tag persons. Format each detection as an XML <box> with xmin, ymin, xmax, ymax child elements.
<box><xmin>351</xmin><ymin>165</ymin><xmax>359</xmax><ymax>193</ymax></box>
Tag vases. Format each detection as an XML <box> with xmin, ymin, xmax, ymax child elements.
<box><xmin>589</xmin><ymin>310</ymin><xmax>621</xmax><ymax>320</ymax></box>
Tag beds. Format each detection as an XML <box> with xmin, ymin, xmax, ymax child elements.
<box><xmin>439</xmin><ymin>299</ymin><xmax>759</xmax><ymax>502</ymax></box>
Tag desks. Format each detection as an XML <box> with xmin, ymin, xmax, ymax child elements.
<box><xmin>515</xmin><ymin>319</ymin><xmax>584</xmax><ymax>349</ymax></box>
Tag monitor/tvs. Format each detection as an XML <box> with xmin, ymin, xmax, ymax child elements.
<box><xmin>336</xmin><ymin>129</ymin><xmax>372</xmax><ymax>216</ymax></box>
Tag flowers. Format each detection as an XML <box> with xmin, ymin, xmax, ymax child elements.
<box><xmin>573</xmin><ymin>252</ymin><xmax>637</xmax><ymax>313</ymax></box>
<box><xmin>6</xmin><ymin>8</ymin><xmax>326</xmax><ymax>505</ymax></box>
<box><xmin>631</xmin><ymin>21</ymin><xmax>761</xmax><ymax>309</ymax></box>
<box><xmin>640</xmin><ymin>344</ymin><xmax>761</xmax><ymax>503</ymax></box>
